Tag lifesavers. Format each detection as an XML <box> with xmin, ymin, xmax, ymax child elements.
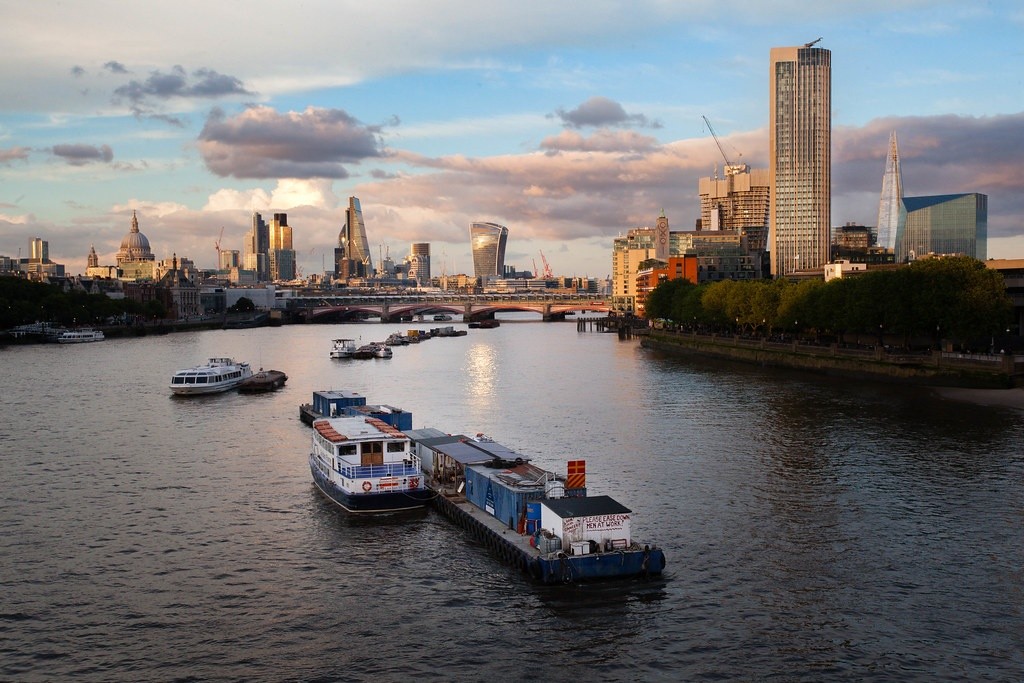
<box><xmin>362</xmin><ymin>480</ymin><xmax>372</xmax><ymax>491</ymax></box>
<box><xmin>410</xmin><ymin>478</ymin><xmax>419</xmax><ymax>487</ymax></box>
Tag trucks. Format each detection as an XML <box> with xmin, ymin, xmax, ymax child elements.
<box><xmin>590</xmin><ymin>302</ymin><xmax>604</xmax><ymax>306</ymax></box>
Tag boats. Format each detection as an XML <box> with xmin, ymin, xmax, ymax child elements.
<box><xmin>467</xmin><ymin>323</ymin><xmax>481</xmax><ymax>328</ymax></box>
<box><xmin>353</xmin><ymin>327</ymin><xmax>467</xmax><ymax>360</ymax></box>
<box><xmin>433</xmin><ymin>315</ymin><xmax>452</xmax><ymax>322</ymax></box>
<box><xmin>238</xmin><ymin>369</ymin><xmax>288</xmax><ymax>393</ymax></box>
<box><xmin>330</xmin><ymin>338</ymin><xmax>356</xmax><ymax>359</ymax></box>
<box><xmin>480</xmin><ymin>319</ymin><xmax>500</xmax><ymax>328</ymax></box>
<box><xmin>307</xmin><ymin>416</ymin><xmax>433</xmax><ymax>519</ymax></box>
<box><xmin>58</xmin><ymin>328</ymin><xmax>105</xmax><ymax>344</ymax></box>
<box><xmin>168</xmin><ymin>357</ymin><xmax>254</xmax><ymax>395</ymax></box>
<box><xmin>299</xmin><ymin>391</ymin><xmax>668</xmax><ymax>588</ymax></box>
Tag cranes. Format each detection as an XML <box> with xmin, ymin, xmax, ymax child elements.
<box><xmin>702</xmin><ymin>116</ymin><xmax>743</xmax><ymax>229</ymax></box>
<box><xmin>214</xmin><ymin>225</ymin><xmax>224</xmax><ymax>268</ymax></box>
